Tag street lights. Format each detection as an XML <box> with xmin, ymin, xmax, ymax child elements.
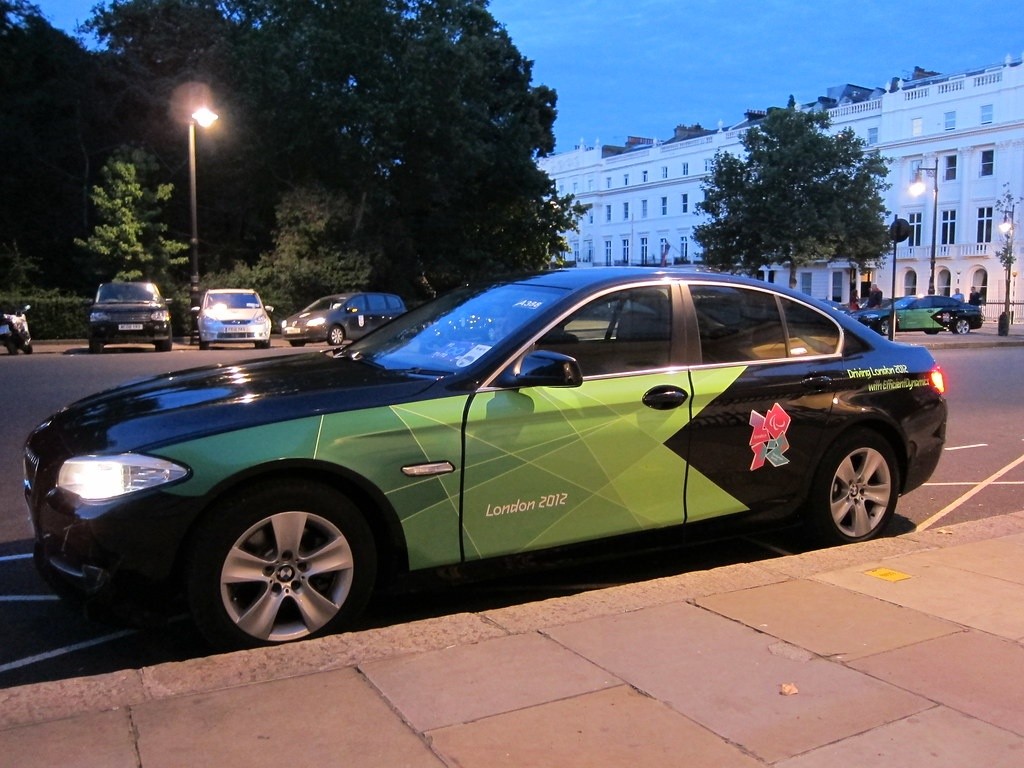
<box><xmin>186</xmin><ymin>102</ymin><xmax>218</xmax><ymax>345</ymax></box>
<box><xmin>998</xmin><ymin>211</ymin><xmax>1014</xmax><ymax>335</ymax></box>
<box><xmin>909</xmin><ymin>156</ymin><xmax>939</xmax><ymax>294</ymax></box>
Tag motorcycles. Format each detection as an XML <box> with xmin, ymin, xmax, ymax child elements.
<box><xmin>0</xmin><ymin>301</ymin><xmax>33</xmax><ymax>355</ymax></box>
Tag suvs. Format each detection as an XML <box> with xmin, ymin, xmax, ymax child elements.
<box><xmin>82</xmin><ymin>279</ymin><xmax>174</xmax><ymax>351</ymax></box>
<box><xmin>281</xmin><ymin>292</ymin><xmax>407</xmax><ymax>346</ymax></box>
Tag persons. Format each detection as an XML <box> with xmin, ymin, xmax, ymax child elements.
<box><xmin>969</xmin><ymin>287</ymin><xmax>981</xmax><ymax>306</ymax></box>
<box><xmin>866</xmin><ymin>284</ymin><xmax>882</xmax><ymax>308</ymax></box>
<box><xmin>952</xmin><ymin>288</ymin><xmax>964</xmax><ymax>303</ymax></box>
<box><xmin>848</xmin><ymin>290</ymin><xmax>859</xmax><ymax>313</ymax></box>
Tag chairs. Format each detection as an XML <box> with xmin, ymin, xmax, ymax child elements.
<box><xmin>616</xmin><ymin>290</ymin><xmax>673</xmax><ymax>368</ymax></box>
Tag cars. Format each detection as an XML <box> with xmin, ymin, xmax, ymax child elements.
<box><xmin>20</xmin><ymin>264</ymin><xmax>949</xmax><ymax>647</ymax></box>
<box><xmin>818</xmin><ymin>297</ymin><xmax>850</xmax><ymax>316</ymax></box>
<box><xmin>190</xmin><ymin>288</ymin><xmax>275</xmax><ymax>350</ymax></box>
<box><xmin>848</xmin><ymin>294</ymin><xmax>985</xmax><ymax>336</ymax></box>
